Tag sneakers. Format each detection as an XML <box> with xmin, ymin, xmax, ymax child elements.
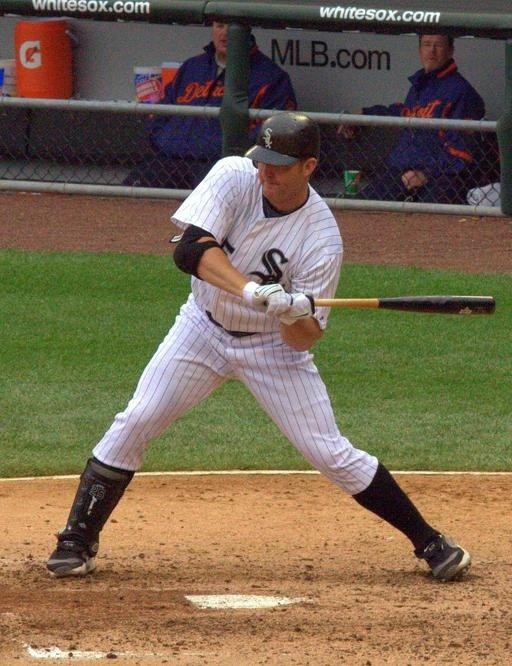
<box><xmin>46</xmin><ymin>528</ymin><xmax>99</xmax><ymax>575</ymax></box>
<box><xmin>414</xmin><ymin>532</ymin><xmax>471</xmax><ymax>581</ymax></box>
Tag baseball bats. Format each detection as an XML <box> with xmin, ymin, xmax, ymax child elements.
<box><xmin>265</xmin><ymin>293</ymin><xmax>496</xmax><ymax>316</ymax></box>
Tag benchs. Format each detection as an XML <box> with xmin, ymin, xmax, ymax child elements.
<box><xmin>0</xmin><ymin>107</ymin><xmax>499</xmax><ymax>205</ymax></box>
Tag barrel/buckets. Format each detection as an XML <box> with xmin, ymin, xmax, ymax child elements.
<box><xmin>13</xmin><ymin>18</ymin><xmax>74</xmax><ymax>97</ymax></box>
<box><xmin>162</xmin><ymin>62</ymin><xmax>182</xmax><ymax>98</ymax></box>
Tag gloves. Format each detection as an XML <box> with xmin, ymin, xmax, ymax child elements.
<box><xmin>278</xmin><ymin>293</ymin><xmax>315</xmax><ymax>326</ymax></box>
<box><xmin>242</xmin><ymin>281</ymin><xmax>292</xmax><ymax>318</ymax></box>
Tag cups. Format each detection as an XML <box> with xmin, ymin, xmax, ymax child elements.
<box><xmin>344</xmin><ymin>169</ymin><xmax>361</xmax><ymax>196</ymax></box>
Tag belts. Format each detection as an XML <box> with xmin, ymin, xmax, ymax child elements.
<box><xmin>206</xmin><ymin>310</ymin><xmax>256</xmax><ymax>338</ymax></box>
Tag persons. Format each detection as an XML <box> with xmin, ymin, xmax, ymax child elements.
<box><xmin>45</xmin><ymin>113</ymin><xmax>470</xmax><ymax>579</ymax></box>
<box><xmin>337</xmin><ymin>34</ymin><xmax>486</xmax><ymax>205</ymax></box>
<box><xmin>122</xmin><ymin>22</ymin><xmax>298</xmax><ymax>190</ymax></box>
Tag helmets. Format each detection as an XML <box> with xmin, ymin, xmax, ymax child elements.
<box><xmin>244</xmin><ymin>112</ymin><xmax>321</xmax><ymax>167</ymax></box>
